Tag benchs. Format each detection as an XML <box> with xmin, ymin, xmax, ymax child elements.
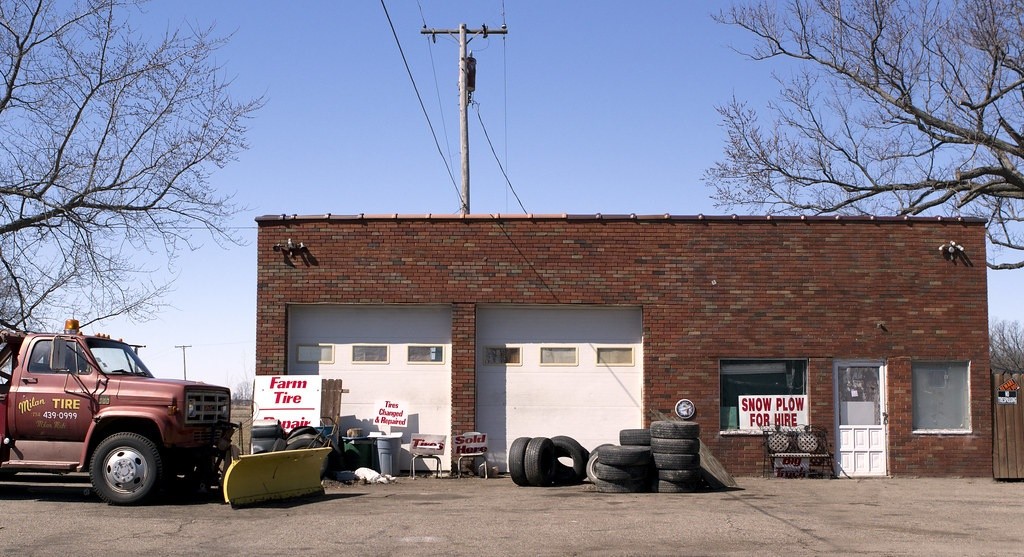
<box><xmin>761</xmin><ymin>423</ymin><xmax>837</xmax><ymax>479</ymax></box>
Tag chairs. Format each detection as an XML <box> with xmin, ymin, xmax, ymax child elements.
<box><xmin>408</xmin><ymin>453</ymin><xmax>441</xmax><ymax>478</ymax></box>
<box><xmin>458</xmin><ymin>432</ymin><xmax>488</xmax><ymax>480</ymax></box>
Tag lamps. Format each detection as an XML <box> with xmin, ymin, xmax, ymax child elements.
<box><xmin>271</xmin><ymin>236</ymin><xmax>305</xmax><ymax>255</ymax></box>
<box><xmin>939</xmin><ymin>240</ymin><xmax>964</xmax><ymax>260</ymax></box>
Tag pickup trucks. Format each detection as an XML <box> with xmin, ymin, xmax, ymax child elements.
<box><xmin>1</xmin><ymin>318</ymin><xmax>235</xmax><ymax>507</ymax></box>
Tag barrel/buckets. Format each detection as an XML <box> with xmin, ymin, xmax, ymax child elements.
<box><xmin>343</xmin><ymin>436</ymin><xmax>375</xmax><ymax>472</ymax></box>
<box><xmin>376</xmin><ymin>437</ymin><xmax>402</xmax><ymax>476</ymax></box>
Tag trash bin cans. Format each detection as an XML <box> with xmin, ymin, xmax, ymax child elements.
<box><xmin>343</xmin><ymin>434</ymin><xmax>374</xmax><ymax>472</ymax></box>
<box><xmin>371</xmin><ymin>433</ymin><xmax>402</xmax><ymax>477</ymax></box>
